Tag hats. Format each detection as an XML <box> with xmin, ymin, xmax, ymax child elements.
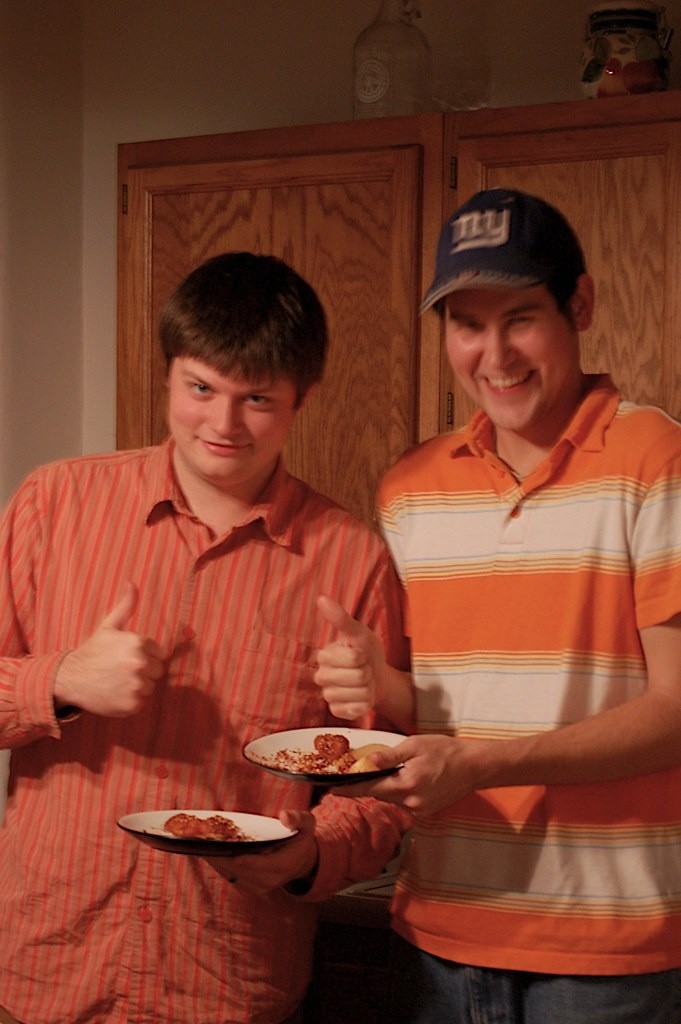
<box><xmin>418</xmin><ymin>188</ymin><xmax>585</xmax><ymax>315</ymax></box>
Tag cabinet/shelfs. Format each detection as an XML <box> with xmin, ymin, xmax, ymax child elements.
<box><xmin>114</xmin><ymin>89</ymin><xmax>681</xmax><ymax>1024</ymax></box>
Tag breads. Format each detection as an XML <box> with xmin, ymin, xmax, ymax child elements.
<box><xmin>315</xmin><ymin>733</ymin><xmax>349</xmax><ymax>761</ymax></box>
<box><xmin>165</xmin><ymin>813</ymin><xmax>238</xmax><ymax>840</ymax></box>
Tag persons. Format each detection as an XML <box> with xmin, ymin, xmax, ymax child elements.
<box><xmin>0</xmin><ymin>246</ymin><xmax>415</xmax><ymax>1023</ymax></box>
<box><xmin>312</xmin><ymin>183</ymin><xmax>680</xmax><ymax>1024</ymax></box>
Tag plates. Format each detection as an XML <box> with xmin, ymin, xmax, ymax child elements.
<box><xmin>116</xmin><ymin>810</ymin><xmax>301</xmax><ymax>858</ymax></box>
<box><xmin>240</xmin><ymin>724</ymin><xmax>414</xmax><ymax>787</ymax></box>
